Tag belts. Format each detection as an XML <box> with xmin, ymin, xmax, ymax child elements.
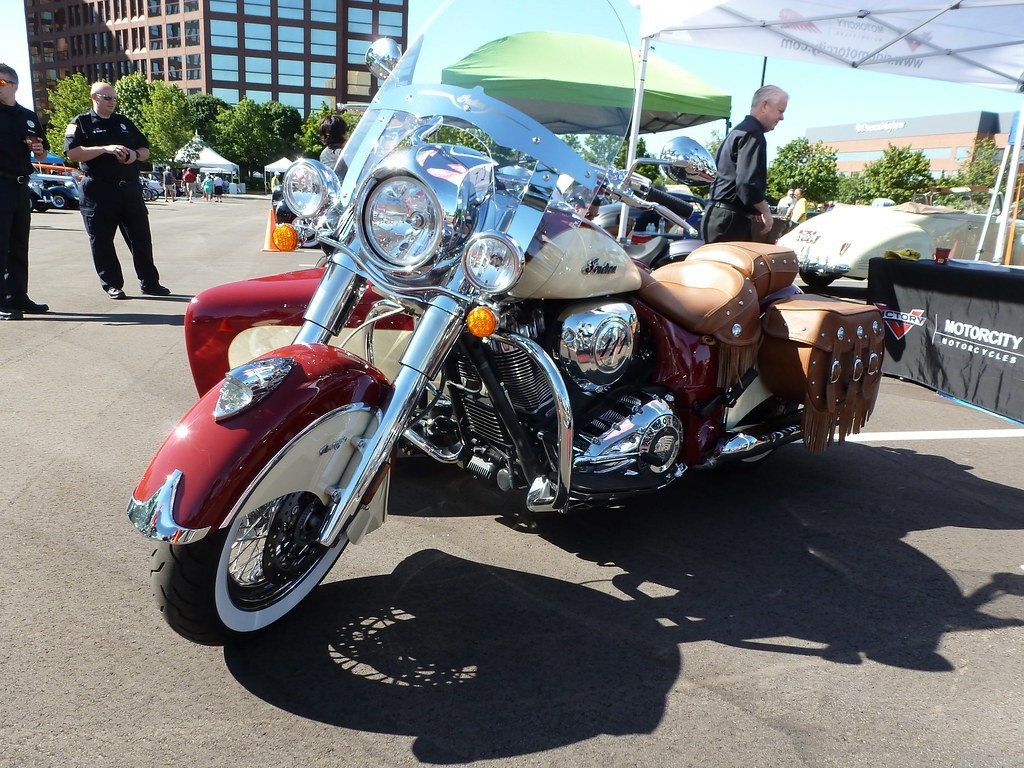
<box><xmin>0</xmin><ymin>174</ymin><xmax>31</xmax><ymax>187</ymax></box>
<box><xmin>708</xmin><ymin>201</ymin><xmax>742</xmax><ymax>211</ymax></box>
<box><xmin>87</xmin><ymin>173</ymin><xmax>138</xmax><ymax>186</ymax></box>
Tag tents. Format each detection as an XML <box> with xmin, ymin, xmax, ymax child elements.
<box><xmin>264</xmin><ymin>157</ymin><xmax>292</xmax><ymax>193</ymax></box>
<box><xmin>615</xmin><ymin>1</ymin><xmax>1023</xmax><ymax>260</ymax></box>
<box><xmin>31</xmin><ymin>151</ymin><xmax>67</xmax><ymax>175</ymax></box>
<box><xmin>442</xmin><ymin>31</ymin><xmax>732</xmax><ymax>138</ymax></box>
<box><xmin>152</xmin><ymin>129</ymin><xmax>241</xmax><ymax>183</ymax></box>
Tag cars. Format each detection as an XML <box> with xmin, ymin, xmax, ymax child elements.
<box><xmin>28</xmin><ymin>162</ymin><xmax>83</xmax><ymax>212</ymax></box>
<box><xmin>138</xmin><ymin>168</ymin><xmax>186</xmax><ymax>201</ymax></box>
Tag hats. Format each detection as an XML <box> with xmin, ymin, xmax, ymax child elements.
<box><xmin>274</xmin><ymin>171</ymin><xmax>281</xmax><ymax>176</ymax></box>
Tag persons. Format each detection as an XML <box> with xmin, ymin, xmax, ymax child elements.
<box><xmin>0</xmin><ymin>64</ymin><xmax>50</xmax><ymax>320</ymax></box>
<box><xmin>777</xmin><ymin>189</ymin><xmax>797</xmax><ymax>214</ymax></box>
<box><xmin>64</xmin><ymin>81</ymin><xmax>171</xmax><ymax>299</ymax></box>
<box><xmin>51</xmin><ymin>163</ymin><xmax>58</xmax><ymax>175</ymax></box>
<box><xmin>701</xmin><ymin>85</ymin><xmax>788</xmax><ymax>242</ymax></box>
<box><xmin>272</xmin><ymin>171</ymin><xmax>280</xmax><ymax>188</ymax></box>
<box><xmin>162</xmin><ymin>165</ymin><xmax>178</xmax><ymax>202</ymax></box>
<box><xmin>786</xmin><ymin>189</ymin><xmax>807</xmax><ymax>227</ymax></box>
<box><xmin>320</xmin><ymin>116</ymin><xmax>349</xmax><ymax>168</ymax></box>
<box><xmin>181</xmin><ymin>168</ymin><xmax>195</xmax><ymax>203</ymax></box>
<box><xmin>826</xmin><ymin>201</ymin><xmax>835</xmax><ymax>211</ymax></box>
<box><xmin>196</xmin><ymin>173</ymin><xmax>223</xmax><ymax>203</ymax></box>
<box><xmin>175</xmin><ymin>169</ymin><xmax>181</xmax><ymax>180</ymax></box>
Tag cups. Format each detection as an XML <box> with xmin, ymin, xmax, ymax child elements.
<box><xmin>935</xmin><ymin>247</ymin><xmax>951</xmax><ymax>264</ymax></box>
<box><xmin>26</xmin><ymin>135</ymin><xmax>38</xmax><ymax>150</ymax></box>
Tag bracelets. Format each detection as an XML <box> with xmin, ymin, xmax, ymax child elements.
<box><xmin>35</xmin><ymin>150</ymin><xmax>44</xmax><ymax>156</ymax></box>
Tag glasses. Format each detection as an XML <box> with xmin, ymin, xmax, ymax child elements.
<box><xmin>0</xmin><ymin>79</ymin><xmax>16</xmax><ymax>86</ymax></box>
<box><xmin>96</xmin><ymin>93</ymin><xmax>117</xmax><ymax>101</ymax></box>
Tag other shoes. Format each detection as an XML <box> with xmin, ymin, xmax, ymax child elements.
<box><xmin>173</xmin><ymin>199</ymin><xmax>178</xmax><ymax>202</ymax></box>
<box><xmin>107</xmin><ymin>287</ymin><xmax>126</xmax><ymax>299</ymax></box>
<box><xmin>20</xmin><ymin>295</ymin><xmax>50</xmax><ymax>314</ymax></box>
<box><xmin>0</xmin><ymin>299</ymin><xmax>24</xmax><ymax>321</ymax></box>
<box><xmin>165</xmin><ymin>200</ymin><xmax>168</xmax><ymax>202</ymax></box>
<box><xmin>142</xmin><ymin>283</ymin><xmax>171</xmax><ymax>296</ymax></box>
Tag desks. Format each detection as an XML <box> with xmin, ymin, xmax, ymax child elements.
<box><xmin>867</xmin><ymin>256</ymin><xmax>1024</xmax><ymax>425</ymax></box>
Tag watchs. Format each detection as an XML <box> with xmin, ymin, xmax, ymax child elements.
<box><xmin>135</xmin><ymin>150</ymin><xmax>140</xmax><ymax>160</ymax></box>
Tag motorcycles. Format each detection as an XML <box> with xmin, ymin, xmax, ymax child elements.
<box><xmin>128</xmin><ymin>0</ymin><xmax>890</xmax><ymax>648</ymax></box>
